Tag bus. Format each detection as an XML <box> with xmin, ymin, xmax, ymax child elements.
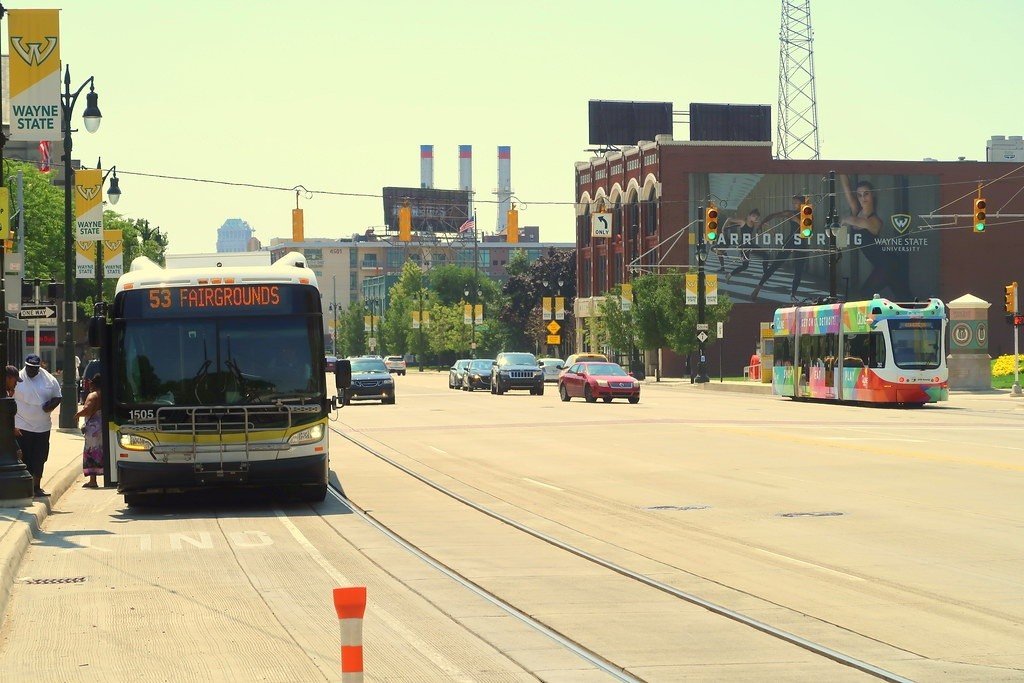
<box><xmin>86</xmin><ymin>251</ymin><xmax>332</xmax><ymax>504</ymax></box>
<box><xmin>772</xmin><ymin>294</ymin><xmax>951</xmax><ymax>410</ymax></box>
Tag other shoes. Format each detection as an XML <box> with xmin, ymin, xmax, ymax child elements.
<box><xmin>82</xmin><ymin>483</ymin><xmax>98</xmax><ymax>487</ymax></box>
<box><xmin>725</xmin><ymin>273</ymin><xmax>731</xmax><ymax>284</ymax></box>
<box><xmin>35</xmin><ymin>489</ymin><xmax>50</xmax><ymax>496</ymax></box>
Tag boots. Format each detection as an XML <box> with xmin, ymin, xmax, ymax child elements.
<box><xmin>790</xmin><ymin>290</ymin><xmax>799</xmax><ymax>302</ymax></box>
<box><xmin>751</xmin><ymin>286</ymin><xmax>762</xmax><ymax>298</ymax></box>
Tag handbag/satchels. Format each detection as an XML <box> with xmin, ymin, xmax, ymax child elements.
<box><xmin>81</xmin><ymin>423</ymin><xmax>87</xmax><ymax>434</ymax></box>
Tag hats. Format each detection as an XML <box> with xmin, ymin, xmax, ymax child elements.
<box><xmin>6</xmin><ymin>365</ymin><xmax>24</xmax><ymax>382</ymax></box>
<box><xmin>26</xmin><ymin>355</ymin><xmax>41</xmax><ymax>367</ymax></box>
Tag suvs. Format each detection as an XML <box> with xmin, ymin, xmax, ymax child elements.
<box><xmin>337</xmin><ymin>358</ymin><xmax>395</xmax><ymax>405</ymax></box>
<box><xmin>489</xmin><ymin>352</ymin><xmax>545</xmax><ymax>395</ymax></box>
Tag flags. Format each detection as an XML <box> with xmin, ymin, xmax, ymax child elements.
<box><xmin>460</xmin><ymin>215</ymin><xmax>474</xmax><ymax>233</ymax></box>
<box><xmin>38</xmin><ymin>141</ymin><xmax>50</xmax><ymax>172</ymax></box>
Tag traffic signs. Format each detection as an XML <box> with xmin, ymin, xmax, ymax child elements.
<box><xmin>592</xmin><ymin>213</ymin><xmax>613</xmax><ymax>239</ymax></box>
<box><xmin>17</xmin><ymin>304</ymin><xmax>56</xmax><ymax>320</ymax></box>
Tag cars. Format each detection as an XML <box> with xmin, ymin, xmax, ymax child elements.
<box><xmin>559</xmin><ymin>361</ymin><xmax>640</xmax><ymax>404</ymax></box>
<box><xmin>79</xmin><ymin>359</ymin><xmax>100</xmax><ymax>404</ymax></box>
<box><xmin>326</xmin><ymin>355</ymin><xmax>407</xmax><ymax>376</ymax></box>
<box><xmin>449</xmin><ymin>358</ymin><xmax>494</xmax><ymax>392</ymax></box>
<box><xmin>538</xmin><ymin>353</ymin><xmax>609</xmax><ymax>382</ymax></box>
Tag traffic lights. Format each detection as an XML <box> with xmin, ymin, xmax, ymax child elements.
<box><xmin>800</xmin><ymin>204</ymin><xmax>813</xmax><ymax>239</ymax></box>
<box><xmin>974</xmin><ymin>199</ymin><xmax>985</xmax><ymax>232</ymax></box>
<box><xmin>705</xmin><ymin>206</ymin><xmax>718</xmax><ymax>242</ymax></box>
<box><xmin>1003</xmin><ymin>284</ymin><xmax>1015</xmax><ymax>313</ymax></box>
<box><xmin>1013</xmin><ymin>315</ymin><xmax>1024</xmax><ymax>326</ymax></box>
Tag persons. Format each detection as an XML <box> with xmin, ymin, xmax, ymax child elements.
<box><xmin>750</xmin><ymin>194</ymin><xmax>808</xmax><ymax>302</ymax></box>
<box><xmin>840</xmin><ymin>174</ymin><xmax>900</xmax><ymax>298</ymax></box>
<box><xmin>711</xmin><ymin>211</ymin><xmax>730</xmax><ymax>271</ymax></box>
<box><xmin>73</xmin><ymin>372</ymin><xmax>104</xmax><ymax>488</ymax></box>
<box><xmin>13</xmin><ymin>352</ymin><xmax>62</xmax><ymax>496</ymax></box>
<box><xmin>722</xmin><ymin>209</ymin><xmax>760</xmax><ymax>280</ymax></box>
<box><xmin>4</xmin><ymin>366</ymin><xmax>23</xmax><ymax>461</ymax></box>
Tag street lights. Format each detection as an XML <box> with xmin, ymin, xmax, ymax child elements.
<box><xmin>824</xmin><ymin>171</ymin><xmax>841</xmax><ymax>298</ymax></box>
<box><xmin>51</xmin><ymin>63</ymin><xmax>103</xmax><ymax>428</ymax></box>
<box><xmin>364</xmin><ymin>295</ymin><xmax>378</xmax><ymax>354</ymax></box>
<box><xmin>542</xmin><ymin>274</ymin><xmax>564</xmax><ymax>358</ymax></box>
<box><xmin>464</xmin><ymin>280</ymin><xmax>483</xmax><ymax>359</ymax></box>
<box><xmin>412</xmin><ymin>288</ymin><xmax>429</xmax><ymax>370</ymax></box>
<box><xmin>694</xmin><ymin>200</ymin><xmax>708</xmax><ymax>384</ymax></box>
<box><xmin>82</xmin><ymin>157</ymin><xmax>121</xmax><ymax>302</ymax></box>
<box><xmin>328</xmin><ymin>274</ymin><xmax>343</xmax><ymax>356</ymax></box>
